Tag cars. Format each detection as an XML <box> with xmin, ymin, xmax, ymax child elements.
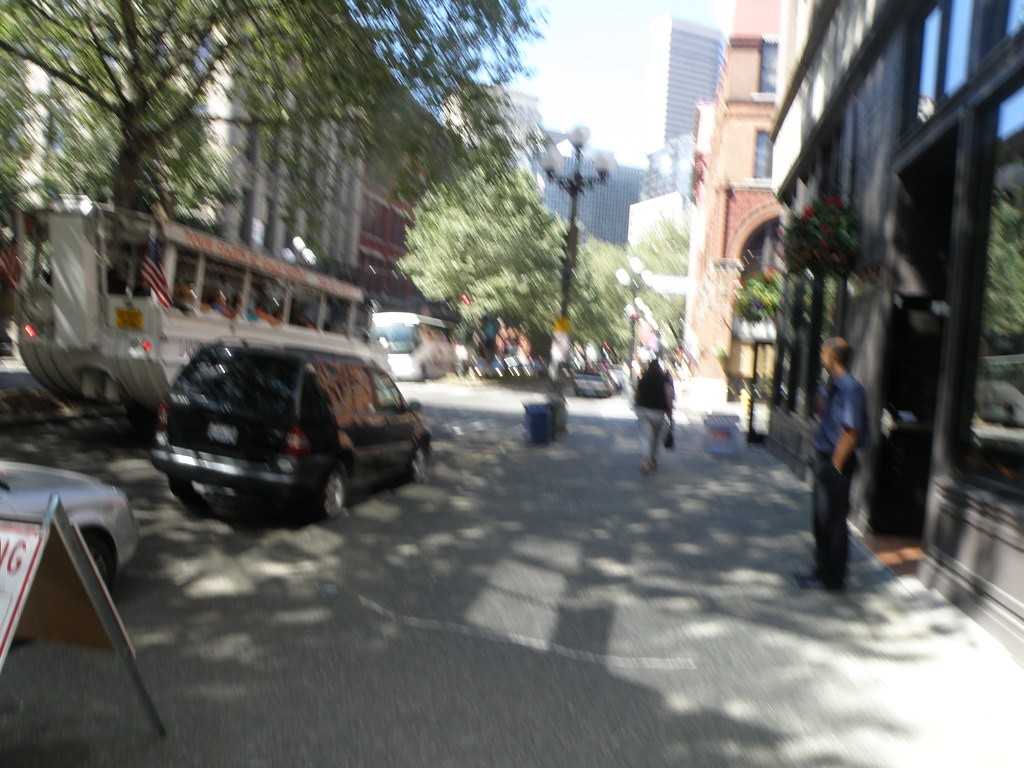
<box><xmin>574</xmin><ymin>362</ymin><xmax>628</xmax><ymax>398</ymax></box>
<box><xmin>150</xmin><ymin>337</ymin><xmax>431</xmax><ymax>520</ymax></box>
<box><xmin>0</xmin><ymin>461</ymin><xmax>141</xmax><ymax>593</ymax></box>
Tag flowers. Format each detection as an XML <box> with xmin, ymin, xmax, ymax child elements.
<box><xmin>778</xmin><ymin>195</ymin><xmax>864</xmax><ymax>279</ymax></box>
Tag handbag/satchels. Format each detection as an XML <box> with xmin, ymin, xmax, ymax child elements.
<box><xmin>663</xmin><ymin>422</ymin><xmax>674</xmax><ymax>449</ymax></box>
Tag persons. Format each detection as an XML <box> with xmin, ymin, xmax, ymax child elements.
<box><xmin>791</xmin><ymin>338</ymin><xmax>866</xmax><ymax>591</ymax></box>
<box><xmin>634</xmin><ymin>359</ymin><xmax>674</xmax><ymax>471</ymax></box>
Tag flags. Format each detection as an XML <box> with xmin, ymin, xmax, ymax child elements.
<box><xmin>139</xmin><ymin>227</ymin><xmax>178</xmax><ymax>311</ymax></box>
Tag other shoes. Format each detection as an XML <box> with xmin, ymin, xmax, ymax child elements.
<box><xmin>639</xmin><ymin>461</ymin><xmax>658</xmax><ymax>476</ymax></box>
<box><xmin>791</xmin><ymin>570</ymin><xmax>843</xmax><ymax>592</ymax></box>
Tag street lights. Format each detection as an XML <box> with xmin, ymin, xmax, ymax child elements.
<box><xmin>540</xmin><ymin>127</ymin><xmax>617</xmax><ymax>432</ymax></box>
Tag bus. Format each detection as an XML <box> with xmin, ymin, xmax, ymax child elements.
<box><xmin>372</xmin><ymin>312</ymin><xmax>459</xmax><ymax>381</ymax></box>
<box><xmin>17</xmin><ymin>197</ymin><xmax>373</xmax><ymax>416</ymax></box>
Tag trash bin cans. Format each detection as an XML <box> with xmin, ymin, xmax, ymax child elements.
<box><xmin>524</xmin><ymin>404</ymin><xmax>555</xmax><ymax>446</ymax></box>
